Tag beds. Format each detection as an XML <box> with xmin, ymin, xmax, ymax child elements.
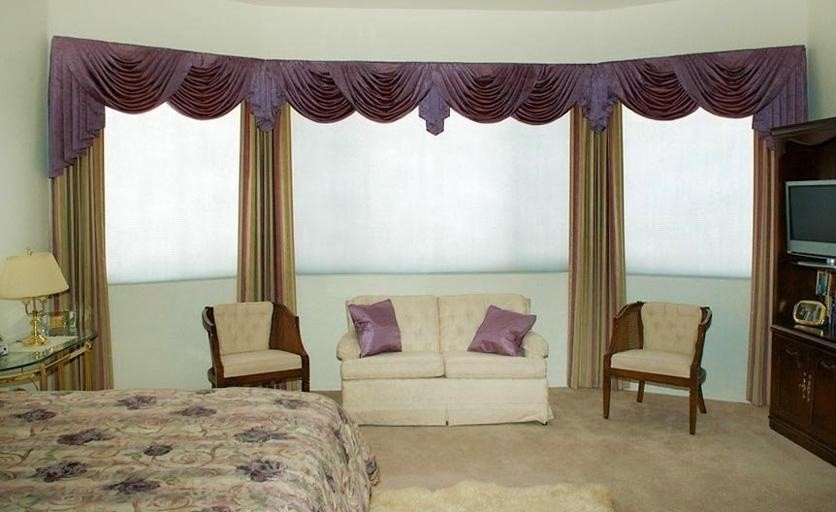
<box><xmin>0</xmin><ymin>391</ymin><xmax>381</xmax><ymax>512</ymax></box>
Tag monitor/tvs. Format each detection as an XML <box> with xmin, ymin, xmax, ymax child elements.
<box><xmin>784</xmin><ymin>180</ymin><xmax>836</xmax><ymax>269</ymax></box>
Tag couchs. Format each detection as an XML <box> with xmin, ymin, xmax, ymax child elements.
<box><xmin>337</xmin><ymin>293</ymin><xmax>554</xmax><ymax>426</ymax></box>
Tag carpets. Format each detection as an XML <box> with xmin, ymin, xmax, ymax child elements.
<box><xmin>366</xmin><ymin>479</ymin><xmax>617</xmax><ymax>511</ymax></box>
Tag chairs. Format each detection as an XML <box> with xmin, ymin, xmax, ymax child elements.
<box><xmin>601</xmin><ymin>301</ymin><xmax>712</xmax><ymax>435</ymax></box>
<box><xmin>201</xmin><ymin>301</ymin><xmax>310</xmax><ymax>393</ymax></box>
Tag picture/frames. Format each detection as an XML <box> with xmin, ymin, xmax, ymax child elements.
<box><xmin>793</xmin><ymin>300</ymin><xmax>827</xmax><ymax>328</ymax></box>
<box><xmin>815</xmin><ymin>270</ymin><xmax>832</xmax><ymax>297</ymax></box>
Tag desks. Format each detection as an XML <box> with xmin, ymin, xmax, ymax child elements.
<box><xmin>0</xmin><ymin>326</ymin><xmax>99</xmax><ymax>391</ymax></box>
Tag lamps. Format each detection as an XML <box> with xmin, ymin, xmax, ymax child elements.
<box><xmin>0</xmin><ymin>247</ymin><xmax>70</xmax><ymax>346</ymax></box>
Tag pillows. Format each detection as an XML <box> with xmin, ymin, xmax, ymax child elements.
<box><xmin>467</xmin><ymin>305</ymin><xmax>536</xmax><ymax>358</ymax></box>
<box><xmin>348</xmin><ymin>299</ymin><xmax>402</xmax><ymax>359</ymax></box>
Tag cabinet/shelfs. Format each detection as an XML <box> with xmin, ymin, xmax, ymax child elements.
<box><xmin>768</xmin><ymin>324</ymin><xmax>836</xmax><ymax>468</ymax></box>
